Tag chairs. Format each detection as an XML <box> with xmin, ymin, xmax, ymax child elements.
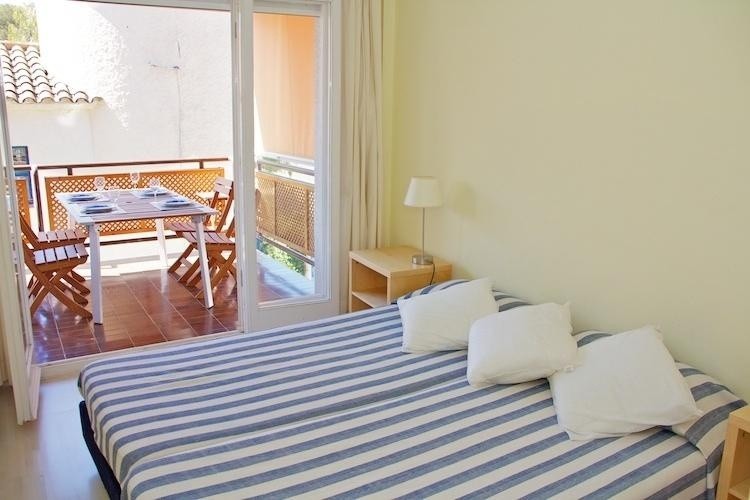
<box><xmin>20</xmin><ymin>211</ymin><xmax>90</xmax><ymax>295</ymax></box>
<box><xmin>182</xmin><ymin>188</ymin><xmax>261</xmax><ymax>298</ymax></box>
<box><xmin>22</xmin><ymin>239</ymin><xmax>93</xmax><ymax>321</ymax></box>
<box><xmin>165</xmin><ymin>177</ymin><xmax>235</xmax><ymax>284</ymax></box>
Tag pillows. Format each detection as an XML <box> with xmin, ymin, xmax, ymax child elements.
<box><xmin>548</xmin><ymin>325</ymin><xmax>702</xmax><ymax>443</ymax></box>
<box><xmin>466</xmin><ymin>300</ymin><xmax>578</xmax><ymax>387</ymax></box>
<box><xmin>397</xmin><ymin>278</ymin><xmax>500</xmax><ymax>354</ymax></box>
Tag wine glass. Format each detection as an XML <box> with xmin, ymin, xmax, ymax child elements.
<box><xmin>108</xmin><ymin>186</ymin><xmax>120</xmax><ymax>210</ymax></box>
<box><xmin>150</xmin><ymin>179</ymin><xmax>160</xmax><ymax>202</ymax></box>
<box><xmin>130</xmin><ymin>171</ymin><xmax>139</xmax><ymax>192</ymax></box>
<box><xmin>94</xmin><ymin>177</ymin><xmax>105</xmax><ymax>198</ymax></box>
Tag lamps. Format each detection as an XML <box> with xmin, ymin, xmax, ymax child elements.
<box><xmin>404</xmin><ymin>177</ymin><xmax>441</xmax><ymax>265</ymax></box>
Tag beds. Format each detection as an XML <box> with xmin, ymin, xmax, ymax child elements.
<box><xmin>78</xmin><ymin>277</ymin><xmax>749</xmax><ymax>500</ymax></box>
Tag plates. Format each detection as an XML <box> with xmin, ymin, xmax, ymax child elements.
<box><xmin>82</xmin><ymin>204</ymin><xmax>113</xmax><ymax>210</ymax></box>
<box><xmin>143</xmin><ymin>190</ymin><xmax>169</xmax><ymax>195</ymax></box>
<box><xmin>68</xmin><ymin>197</ymin><xmax>95</xmax><ymax>201</ymax></box>
<box><xmin>161</xmin><ymin>201</ymin><xmax>192</xmax><ymax>207</ymax></box>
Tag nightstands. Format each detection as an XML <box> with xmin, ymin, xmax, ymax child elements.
<box><xmin>348</xmin><ymin>245</ymin><xmax>452</xmax><ymax>313</ymax></box>
<box><xmin>714</xmin><ymin>403</ymin><xmax>750</xmax><ymax>500</ymax></box>
